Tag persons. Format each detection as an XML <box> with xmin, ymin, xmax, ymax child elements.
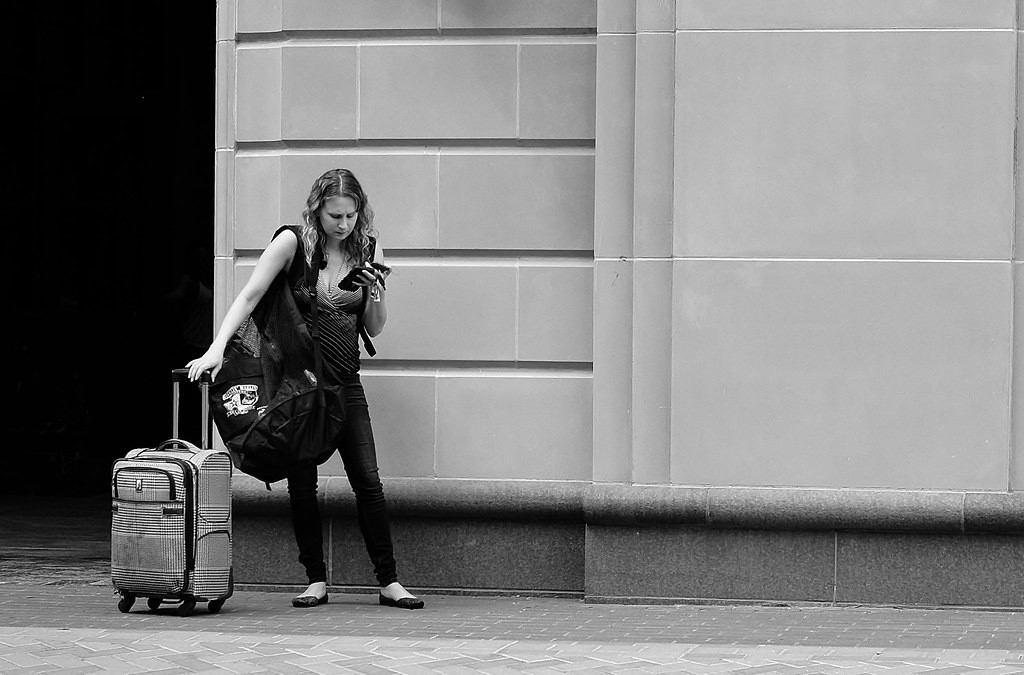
<box><xmin>184</xmin><ymin>169</ymin><xmax>425</xmax><ymax>609</ymax></box>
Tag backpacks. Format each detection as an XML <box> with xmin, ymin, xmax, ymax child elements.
<box><xmin>199</xmin><ymin>225</ymin><xmax>376</xmax><ymax>490</ymax></box>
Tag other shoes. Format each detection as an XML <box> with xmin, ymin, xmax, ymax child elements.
<box><xmin>292</xmin><ymin>592</ymin><xmax>328</xmax><ymax>607</ymax></box>
<box><xmin>379</xmin><ymin>591</ymin><xmax>424</xmax><ymax>609</ymax></box>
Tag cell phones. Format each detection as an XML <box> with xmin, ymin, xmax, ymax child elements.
<box><xmin>338</xmin><ymin>266</ymin><xmax>374</xmax><ymax>292</ymax></box>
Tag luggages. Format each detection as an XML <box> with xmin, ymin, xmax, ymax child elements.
<box><xmin>110</xmin><ymin>369</ymin><xmax>232</xmax><ymax>617</ymax></box>
